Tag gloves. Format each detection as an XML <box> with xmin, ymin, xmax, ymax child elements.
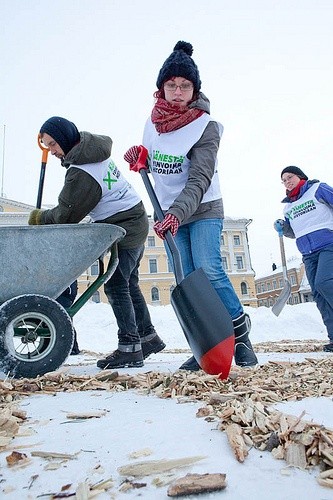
<box><xmin>273</xmin><ymin>219</ymin><xmax>283</xmax><ymax>232</ymax></box>
<box><xmin>124</xmin><ymin>145</ymin><xmax>140</xmax><ymax>162</ymax></box>
<box><xmin>28</xmin><ymin>208</ymin><xmax>45</xmax><ymax>225</ymax></box>
<box><xmin>153</xmin><ymin>214</ymin><xmax>179</xmax><ymax>241</ymax></box>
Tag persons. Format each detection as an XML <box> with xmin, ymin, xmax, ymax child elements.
<box><xmin>273</xmin><ymin>166</ymin><xmax>333</xmax><ymax>353</ymax></box>
<box><xmin>125</xmin><ymin>41</ymin><xmax>259</xmax><ymax>371</ymax></box>
<box><xmin>28</xmin><ymin>116</ymin><xmax>166</xmax><ymax>369</ymax></box>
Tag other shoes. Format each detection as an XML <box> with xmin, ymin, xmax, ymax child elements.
<box><xmin>323</xmin><ymin>342</ymin><xmax>333</xmax><ymax>352</ymax></box>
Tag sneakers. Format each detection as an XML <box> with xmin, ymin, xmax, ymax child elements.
<box><xmin>141</xmin><ymin>335</ymin><xmax>166</xmax><ymax>358</ymax></box>
<box><xmin>97</xmin><ymin>349</ymin><xmax>144</xmax><ymax>369</ymax></box>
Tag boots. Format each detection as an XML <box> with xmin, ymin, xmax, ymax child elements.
<box><xmin>231</xmin><ymin>312</ymin><xmax>258</xmax><ymax>366</ymax></box>
<box><xmin>179</xmin><ymin>352</ymin><xmax>202</xmax><ymax>371</ymax></box>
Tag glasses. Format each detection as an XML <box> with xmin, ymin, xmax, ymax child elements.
<box><xmin>165</xmin><ymin>81</ymin><xmax>194</xmax><ymax>92</ymax></box>
<box><xmin>281</xmin><ymin>174</ymin><xmax>296</xmax><ymax>184</ymax></box>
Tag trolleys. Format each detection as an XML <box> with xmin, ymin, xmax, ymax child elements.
<box><xmin>0</xmin><ymin>223</ymin><xmax>127</xmax><ymax>373</ymax></box>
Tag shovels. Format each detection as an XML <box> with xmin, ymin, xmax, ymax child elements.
<box><xmin>36</xmin><ymin>136</ymin><xmax>50</xmax><ymax>209</ymax></box>
<box><xmin>271</xmin><ymin>229</ymin><xmax>292</xmax><ymax>316</ymax></box>
<box><xmin>128</xmin><ymin>145</ymin><xmax>235</xmax><ymax>380</ymax></box>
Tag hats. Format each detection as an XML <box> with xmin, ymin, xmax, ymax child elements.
<box><xmin>41</xmin><ymin>118</ymin><xmax>80</xmax><ymax>156</ymax></box>
<box><xmin>281</xmin><ymin>166</ymin><xmax>308</xmax><ymax>180</ymax></box>
<box><xmin>155</xmin><ymin>40</ymin><xmax>202</xmax><ymax>90</ymax></box>
